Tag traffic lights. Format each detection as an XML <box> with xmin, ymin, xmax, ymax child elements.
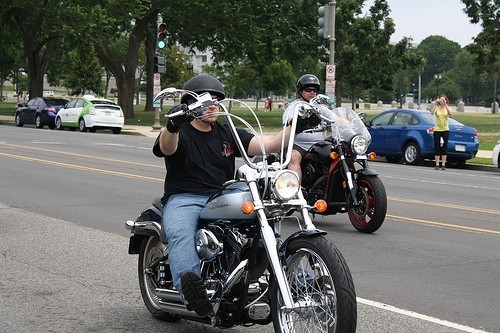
<box><xmin>317</xmin><ymin>5</ymin><xmax>325</xmax><ymax>38</ymax></box>
<box><xmin>157</xmin><ymin>22</ymin><xmax>167</xmax><ymax>49</ymax></box>
<box><xmin>154</xmin><ymin>56</ymin><xmax>165</xmax><ymax>74</ymax></box>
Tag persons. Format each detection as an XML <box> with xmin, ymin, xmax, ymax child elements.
<box><xmin>265</xmin><ymin>97</ymin><xmax>272</xmax><ymax>111</ymax></box>
<box><xmin>431</xmin><ymin>95</ymin><xmax>452</xmax><ymax>170</ymax></box>
<box><xmin>153</xmin><ymin>75</ymin><xmax>322</xmax><ymax>315</ymax></box>
<box><xmin>283</xmin><ymin>74</ymin><xmax>351</xmax><ymax>184</ymax></box>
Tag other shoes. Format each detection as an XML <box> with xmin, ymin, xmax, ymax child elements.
<box><xmin>435</xmin><ymin>166</ymin><xmax>439</xmax><ymax>170</ymax></box>
<box><xmin>296</xmin><ymin>275</ymin><xmax>331</xmax><ymax>300</ymax></box>
<box><xmin>181</xmin><ymin>269</ymin><xmax>213</xmax><ymax>322</ymax></box>
<box><xmin>441</xmin><ymin>166</ymin><xmax>445</xmax><ymax>170</ymax></box>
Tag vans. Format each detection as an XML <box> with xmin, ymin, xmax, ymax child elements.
<box><xmin>42</xmin><ymin>91</ymin><xmax>54</xmax><ymax>97</ymax></box>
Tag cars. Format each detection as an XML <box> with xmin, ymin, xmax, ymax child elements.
<box><xmin>492</xmin><ymin>139</ymin><xmax>500</xmax><ymax>169</ymax></box>
<box><xmin>362</xmin><ymin>108</ymin><xmax>479</xmax><ymax>164</ymax></box>
<box><xmin>15</xmin><ymin>97</ymin><xmax>68</xmax><ymax>129</ymax></box>
<box><xmin>54</xmin><ymin>97</ymin><xmax>124</xmax><ymax>135</ymax></box>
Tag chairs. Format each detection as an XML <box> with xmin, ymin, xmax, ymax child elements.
<box><xmin>401</xmin><ymin>116</ymin><xmax>409</xmax><ymax>123</ymax></box>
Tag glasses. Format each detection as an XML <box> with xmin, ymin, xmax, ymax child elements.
<box><xmin>303</xmin><ymin>87</ymin><xmax>317</xmax><ymax>92</ymax></box>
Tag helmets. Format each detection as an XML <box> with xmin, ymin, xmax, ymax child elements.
<box><xmin>297</xmin><ymin>74</ymin><xmax>320</xmax><ymax>92</ymax></box>
<box><xmin>181</xmin><ymin>75</ymin><xmax>226</xmax><ymax>103</ymax></box>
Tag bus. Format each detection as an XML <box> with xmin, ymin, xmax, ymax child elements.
<box><xmin>405</xmin><ymin>93</ymin><xmax>414</xmax><ymax>102</ymax></box>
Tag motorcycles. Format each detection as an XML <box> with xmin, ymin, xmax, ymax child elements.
<box><xmin>125</xmin><ymin>89</ymin><xmax>357</xmax><ymax>333</ymax></box>
<box><xmin>247</xmin><ymin>96</ymin><xmax>387</xmax><ymax>232</ymax></box>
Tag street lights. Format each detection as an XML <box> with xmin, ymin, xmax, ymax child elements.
<box><xmin>434</xmin><ymin>74</ymin><xmax>443</xmax><ymax>99</ymax></box>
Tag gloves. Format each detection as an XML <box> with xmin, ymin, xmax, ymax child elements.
<box><xmin>298</xmin><ymin>113</ymin><xmax>321</xmax><ymax>127</ymax></box>
<box><xmin>167</xmin><ymin>103</ymin><xmax>194</xmax><ymax>129</ymax></box>
